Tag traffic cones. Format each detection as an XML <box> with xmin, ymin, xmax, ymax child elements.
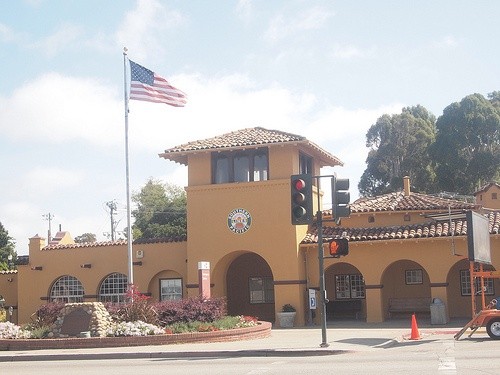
<box><xmin>408</xmin><ymin>314</ymin><xmax>423</xmax><ymax>341</ymax></box>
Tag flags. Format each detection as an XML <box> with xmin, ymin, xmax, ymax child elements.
<box><xmin>126</xmin><ymin>60</ymin><xmax>191</xmax><ymax>108</ymax></box>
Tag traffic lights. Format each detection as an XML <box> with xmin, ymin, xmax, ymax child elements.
<box><xmin>330</xmin><ymin>175</ymin><xmax>351</xmax><ymax>224</ymax></box>
<box><xmin>290</xmin><ymin>174</ymin><xmax>313</xmax><ymax>225</ymax></box>
<box><xmin>329</xmin><ymin>238</ymin><xmax>349</xmax><ymax>256</ymax></box>
